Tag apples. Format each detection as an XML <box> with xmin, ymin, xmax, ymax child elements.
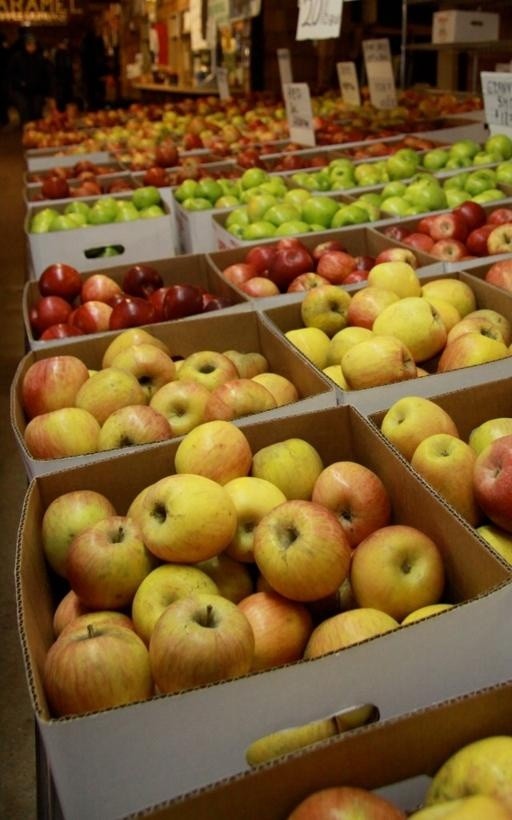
<box><xmin>175</xmin><ymin>135</ymin><xmax>512</xmax><ymax>241</ymax></box>
<box><xmin>22</xmin><ymin>328</ymin><xmax>301</xmax><ymax>461</ymax></box>
<box><xmin>21</xmin><ymin>201</ymin><xmax>512</xmax><ymax>343</ymax></box>
<box><xmin>42</xmin><ymin>419</ymin><xmax>460</xmax><ymax>766</ymax></box>
<box><xmin>29</xmin><ymin>188</ymin><xmax>173</xmax><ymax>257</ymax></box>
<box><xmin>285</xmin><ymin>261</ymin><xmax>512</xmax><ymax>392</ymax></box>
<box><xmin>382</xmin><ymin>396</ymin><xmax>512</xmax><ymax>567</ymax></box>
<box><xmin>281</xmin><ymin>726</ymin><xmax>512</xmax><ymax>817</ymax></box>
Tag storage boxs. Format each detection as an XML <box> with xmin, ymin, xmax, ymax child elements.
<box><xmin>14</xmin><ymin>403</ymin><xmax>511</xmax><ymax>820</ymax></box>
<box><xmin>206</xmin><ymin>222</ymin><xmax>447</xmax><ymax>312</ymax></box>
<box><xmin>273</xmin><ymin>112</ymin><xmax>389</xmax><ymax>236</ymax></box>
<box><xmin>389</xmin><ymin>115</ymin><xmax>512</xmax><ymax>225</ymax></box>
<box><xmin>458</xmin><ymin>254</ymin><xmax>512</xmax><ymax>299</ymax></box>
<box><xmin>20</xmin><ymin>142</ymin><xmax>176</xmax><ymax>279</ymax></box>
<box><xmin>8</xmin><ymin>307</ymin><xmax>338</xmax><ymax>484</ymax></box>
<box><xmin>117</xmin><ymin>138</ymin><xmax>273</xmax><ymax>252</ymax></box>
<box><xmin>369</xmin><ymin>204</ymin><xmax>512</xmax><ymax>273</ymax></box>
<box><xmin>364</xmin><ymin>372</ymin><xmax>512</xmax><ymax>573</ymax></box>
<box><xmin>432</xmin><ymin>9</ymin><xmax>500</xmax><ymax>44</ymax></box>
<box><xmin>20</xmin><ymin>252</ymin><xmax>254</xmax><ymax>349</ymax></box>
<box><xmin>121</xmin><ymin>677</ymin><xmax>511</xmax><ymax>820</ymax></box>
<box><xmin>262</xmin><ymin>270</ymin><xmax>512</xmax><ymax>414</ymax></box>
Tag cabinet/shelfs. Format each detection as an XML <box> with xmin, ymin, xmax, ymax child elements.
<box><xmin>128</xmin><ymin>0</ymin><xmax>246</xmax><ymax>96</ymax></box>
